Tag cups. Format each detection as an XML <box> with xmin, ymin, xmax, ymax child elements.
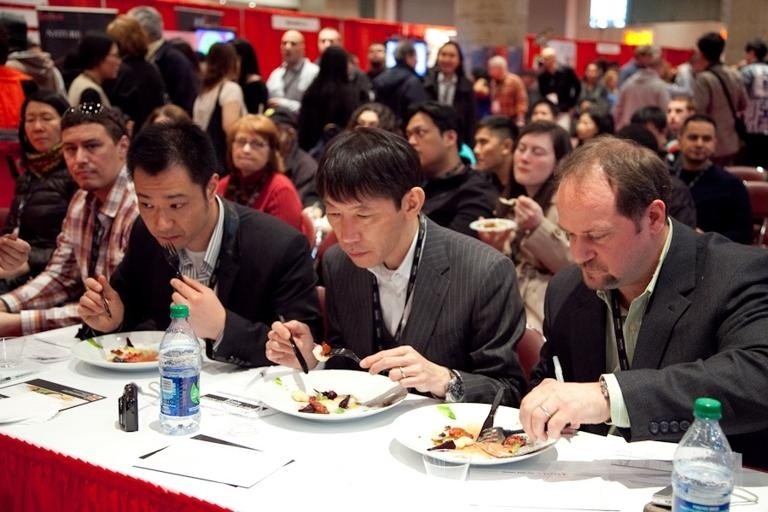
<box><xmin>0</xmin><ymin>335</ymin><xmax>23</xmax><ymax>370</ymax></box>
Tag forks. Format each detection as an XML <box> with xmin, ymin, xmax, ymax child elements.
<box><xmin>325</xmin><ymin>347</ymin><xmax>363</xmax><ymax>368</ymax></box>
<box><xmin>159</xmin><ymin>239</ymin><xmax>183</xmax><ymax>285</ymax></box>
<box><xmin>477</xmin><ymin>424</ymin><xmax>578</xmax><ymax>447</ymax></box>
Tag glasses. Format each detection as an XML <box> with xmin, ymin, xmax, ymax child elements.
<box><xmin>62</xmin><ymin>102</ymin><xmax>126</xmax><ymax>135</ymax></box>
<box><xmin>233</xmin><ymin>137</ymin><xmax>268</xmax><ymax>149</ymax></box>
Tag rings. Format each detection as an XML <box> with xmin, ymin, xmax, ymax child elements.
<box><xmin>399</xmin><ymin>367</ymin><xmax>404</xmax><ymax>379</ymax></box>
<box><xmin>540</xmin><ymin>404</ymin><xmax>551</xmax><ymax>417</ymax></box>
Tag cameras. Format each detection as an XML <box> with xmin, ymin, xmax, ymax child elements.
<box><xmin>118</xmin><ymin>383</ymin><xmax>137</xmax><ymax>433</ymax></box>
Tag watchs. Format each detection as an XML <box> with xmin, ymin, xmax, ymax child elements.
<box><xmin>443</xmin><ymin>368</ymin><xmax>465</xmax><ymax>404</ymax></box>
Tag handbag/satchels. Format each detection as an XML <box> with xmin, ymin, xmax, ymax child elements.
<box><xmin>206</xmin><ymin>104</ymin><xmax>231</xmax><ymax>173</ymax></box>
<box><xmin>735</xmin><ymin>114</ymin><xmax>748</xmax><ymax>140</ymax></box>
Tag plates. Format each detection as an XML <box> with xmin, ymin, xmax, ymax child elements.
<box><xmin>468</xmin><ymin>217</ymin><xmax>517</xmax><ymax>233</ymax></box>
<box><xmin>388</xmin><ymin>401</ymin><xmax>558</xmax><ymax>463</ymax></box>
<box><xmin>257</xmin><ymin>366</ymin><xmax>408</xmax><ymax>421</ymax></box>
<box><xmin>70</xmin><ymin>330</ymin><xmax>207</xmax><ymax>370</ymax></box>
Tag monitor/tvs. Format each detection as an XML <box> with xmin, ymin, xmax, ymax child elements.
<box><xmin>194</xmin><ymin>28</ymin><xmax>236</xmax><ymax>56</ymax></box>
<box><xmin>384</xmin><ymin>39</ymin><xmax>428</xmax><ymax>77</ymax></box>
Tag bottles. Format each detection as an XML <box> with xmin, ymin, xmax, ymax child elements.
<box><xmin>667</xmin><ymin>397</ymin><xmax>734</xmax><ymax>512</ymax></box>
<box><xmin>155</xmin><ymin>303</ymin><xmax>202</xmax><ymax>434</ymax></box>
<box><xmin>117</xmin><ymin>384</ymin><xmax>140</xmax><ymax>431</ymax></box>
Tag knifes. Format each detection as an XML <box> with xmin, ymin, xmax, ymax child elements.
<box><xmin>277</xmin><ymin>313</ymin><xmax>311</xmax><ymax>374</ymax></box>
<box><xmin>97</xmin><ymin>287</ymin><xmax>116</xmax><ymax>319</ymax></box>
<box><xmin>476</xmin><ymin>386</ymin><xmax>506</xmax><ymax>438</ymax></box>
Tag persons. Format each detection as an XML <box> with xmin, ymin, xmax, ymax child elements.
<box><xmin>78</xmin><ymin>120</ymin><xmax>317</xmax><ymax>369</ymax></box>
<box><xmin>518</xmin><ymin>138</ymin><xmax>768</xmax><ymax>444</ymax></box>
<box><xmin>1</xmin><ymin>226</ymin><xmax>32</xmax><ymax>279</ymax></box>
<box><xmin>0</xmin><ymin>6</ymin><xmax>768</xmax><ymax>338</ymax></box>
<box><xmin>264</xmin><ymin>126</ymin><xmax>524</xmax><ymax>406</ymax></box>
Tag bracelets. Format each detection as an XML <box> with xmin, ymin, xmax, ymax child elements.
<box><xmin>599</xmin><ymin>375</ymin><xmax>608</xmax><ymax>408</ymax></box>
